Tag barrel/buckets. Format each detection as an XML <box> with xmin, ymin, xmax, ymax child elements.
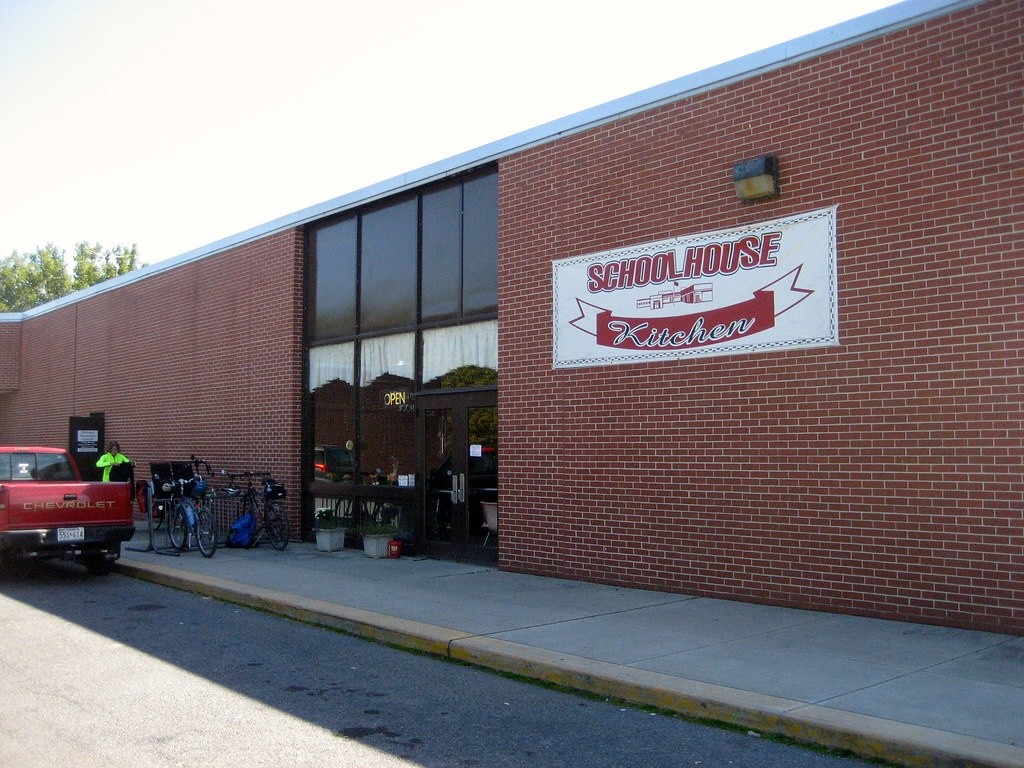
<box><xmin>387</xmin><ymin>540</ymin><xmax>401</xmax><ymax>558</ymax></box>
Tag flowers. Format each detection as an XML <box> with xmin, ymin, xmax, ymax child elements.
<box><xmin>313</xmin><ymin>507</ymin><xmax>342</xmax><ymax>529</ymax></box>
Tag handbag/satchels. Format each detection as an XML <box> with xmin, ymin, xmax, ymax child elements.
<box><xmin>265</xmin><ymin>483</ymin><xmax>286</xmax><ymax>500</ymax></box>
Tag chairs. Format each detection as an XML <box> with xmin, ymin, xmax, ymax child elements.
<box><xmin>149</xmin><ymin>460</ymin><xmax>198</xmax><ymax>513</ymax></box>
<box><xmin>480</xmin><ymin>501</ymin><xmax>498</xmax><ymax>546</ymax></box>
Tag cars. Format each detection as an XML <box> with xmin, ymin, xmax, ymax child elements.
<box><xmin>428</xmin><ymin>447</ymin><xmax>498</xmax><ymax>535</ymax></box>
<box><xmin>314</xmin><ymin>442</ymin><xmax>355</xmax><ymax>483</ymax></box>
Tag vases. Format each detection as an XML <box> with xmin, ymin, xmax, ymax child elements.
<box><xmin>312</xmin><ymin>527</ymin><xmax>347</xmax><ymax>552</ymax></box>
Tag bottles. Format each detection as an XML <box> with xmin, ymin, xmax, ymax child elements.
<box><xmin>187</xmin><ymin>504</ymin><xmax>195</xmax><ymax>526</ymax></box>
<box><xmin>267</xmin><ymin>485</ymin><xmax>272</xmax><ymax>492</ymax></box>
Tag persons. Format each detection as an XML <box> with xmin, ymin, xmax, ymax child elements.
<box><xmin>96</xmin><ymin>440</ymin><xmax>136</xmax><ymax>483</ymax></box>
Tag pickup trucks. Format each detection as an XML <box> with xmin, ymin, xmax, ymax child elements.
<box><xmin>0</xmin><ymin>445</ymin><xmax>136</xmax><ymax>578</ymax></box>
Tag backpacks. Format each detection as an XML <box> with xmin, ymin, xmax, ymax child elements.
<box><xmin>227</xmin><ymin>513</ymin><xmax>255</xmax><ymax>548</ymax></box>
<box><xmin>136</xmin><ymin>479</ymin><xmax>154</xmax><ymax>513</ymax></box>
<box><xmin>175</xmin><ymin>501</ymin><xmax>195</xmax><ymax>534</ymax></box>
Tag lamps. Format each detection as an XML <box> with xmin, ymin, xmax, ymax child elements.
<box><xmin>732</xmin><ymin>154</ymin><xmax>777</xmax><ymax>203</ymax></box>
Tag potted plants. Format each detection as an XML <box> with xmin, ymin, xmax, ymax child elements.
<box><xmin>356</xmin><ymin>519</ymin><xmax>398</xmax><ymax>559</ymax></box>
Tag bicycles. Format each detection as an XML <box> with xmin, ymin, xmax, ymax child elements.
<box><xmin>220</xmin><ymin>468</ymin><xmax>290</xmax><ymax>551</ymax></box>
<box><xmin>136</xmin><ymin>454</ymin><xmax>218</xmax><ymax>558</ymax></box>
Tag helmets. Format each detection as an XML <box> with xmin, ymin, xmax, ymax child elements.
<box><xmin>191</xmin><ymin>480</ymin><xmax>208</xmax><ymax>500</ymax></box>
<box><xmin>223</xmin><ymin>487</ymin><xmax>241</xmax><ymax>497</ymax></box>
